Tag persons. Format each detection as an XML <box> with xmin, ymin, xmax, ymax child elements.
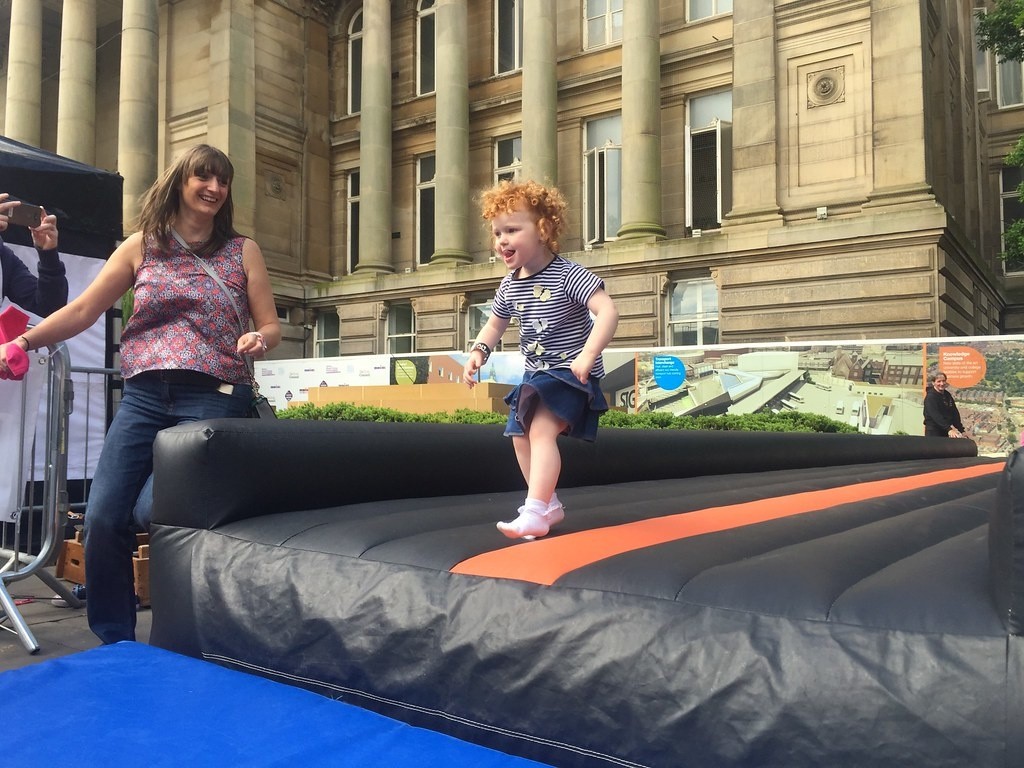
<box><xmin>51</xmin><ymin>525</ymin><xmax>141</xmax><ymax>612</ymax></box>
<box><xmin>461</xmin><ymin>177</ymin><xmax>619</xmax><ymax>540</ymax></box>
<box><xmin>0</xmin><ymin>193</ymin><xmax>69</xmax><ymax>626</ymax></box>
<box><xmin>0</xmin><ymin>144</ymin><xmax>282</xmax><ymax>645</ymax></box>
<box><xmin>922</xmin><ymin>372</ymin><xmax>967</xmax><ymax>439</ymax></box>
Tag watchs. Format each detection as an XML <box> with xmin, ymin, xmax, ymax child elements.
<box><xmin>247</xmin><ymin>331</ymin><xmax>267</xmax><ymax>351</ymax></box>
<box><xmin>470</xmin><ymin>342</ymin><xmax>491</xmax><ymax>365</ymax></box>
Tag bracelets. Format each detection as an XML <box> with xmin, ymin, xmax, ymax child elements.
<box><xmin>17</xmin><ymin>335</ymin><xmax>30</xmax><ymax>353</ymax></box>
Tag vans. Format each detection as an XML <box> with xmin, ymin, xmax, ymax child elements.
<box><xmin>851</xmin><ymin>401</ymin><xmax>860</xmax><ymax>415</ymax></box>
<box><xmin>836</xmin><ymin>401</ymin><xmax>843</xmax><ymax>414</ymax></box>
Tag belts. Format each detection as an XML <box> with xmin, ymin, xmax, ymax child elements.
<box><xmin>132</xmin><ymin>371</ymin><xmax>217</xmax><ymax>387</ymax></box>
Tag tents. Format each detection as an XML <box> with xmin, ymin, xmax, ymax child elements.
<box><xmin>1</xmin><ymin>136</ymin><xmax>125</xmax><ymax>567</ymax></box>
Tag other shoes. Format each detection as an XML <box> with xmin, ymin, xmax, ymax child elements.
<box><xmin>51</xmin><ymin>583</ymin><xmax>86</xmax><ymax>608</ymax></box>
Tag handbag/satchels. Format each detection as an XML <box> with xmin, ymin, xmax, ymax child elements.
<box><xmin>249</xmin><ymin>391</ymin><xmax>279</xmax><ymax>418</ymax></box>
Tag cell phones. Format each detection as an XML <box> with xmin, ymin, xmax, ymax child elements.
<box><xmin>0</xmin><ymin>199</ymin><xmax>42</xmax><ymax>229</ymax></box>
